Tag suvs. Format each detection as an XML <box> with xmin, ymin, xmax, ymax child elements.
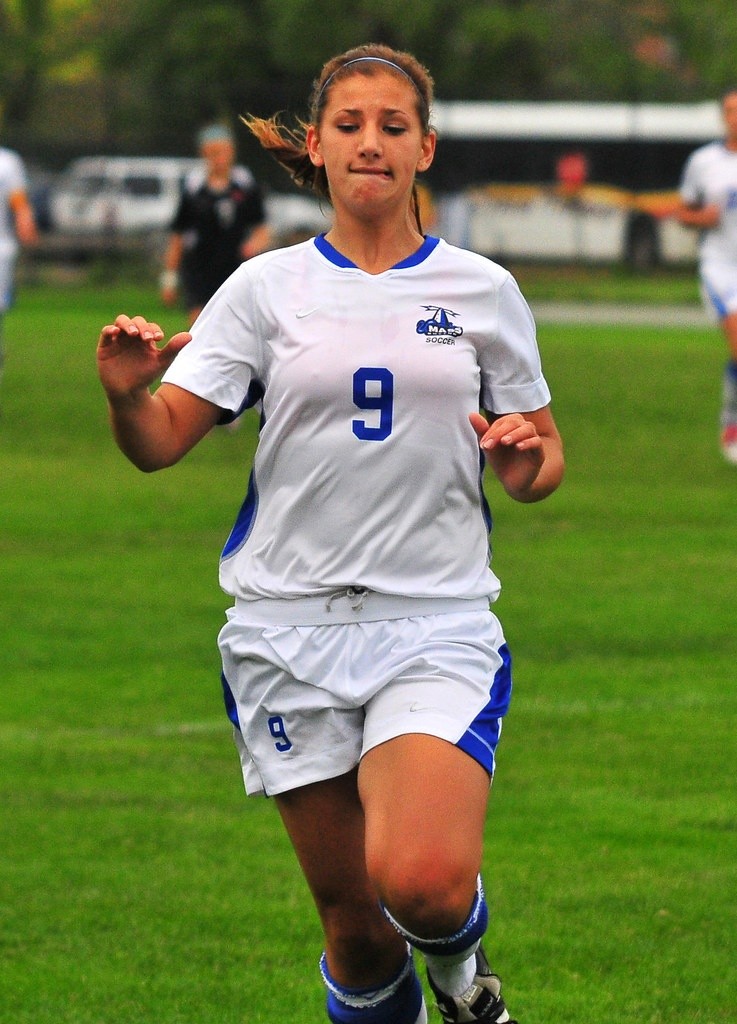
<box><xmin>44</xmin><ymin>156</ymin><xmax>256</xmax><ymax>239</ymax></box>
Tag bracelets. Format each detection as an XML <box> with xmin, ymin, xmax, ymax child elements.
<box><xmin>162</xmin><ymin>271</ymin><xmax>176</xmax><ymax>287</ymax></box>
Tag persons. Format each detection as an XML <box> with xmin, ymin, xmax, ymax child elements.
<box><xmin>98</xmin><ymin>43</ymin><xmax>562</xmax><ymax>1024</ymax></box>
<box><xmin>626</xmin><ymin>86</ymin><xmax>737</xmax><ymax>470</ymax></box>
<box><xmin>160</xmin><ymin>119</ymin><xmax>272</xmax><ymax>431</ymax></box>
<box><xmin>0</xmin><ymin>145</ymin><xmax>38</xmax><ymax>326</ymax></box>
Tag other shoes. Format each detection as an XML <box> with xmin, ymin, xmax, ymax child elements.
<box><xmin>720</xmin><ymin>425</ymin><xmax>737</xmax><ymax>468</ymax></box>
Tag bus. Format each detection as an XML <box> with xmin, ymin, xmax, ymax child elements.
<box><xmin>417</xmin><ymin>94</ymin><xmax>724</xmax><ymax>275</ymax></box>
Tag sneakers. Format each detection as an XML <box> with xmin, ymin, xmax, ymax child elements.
<box><xmin>427</xmin><ymin>946</ymin><xmax>518</xmax><ymax>1024</ymax></box>
